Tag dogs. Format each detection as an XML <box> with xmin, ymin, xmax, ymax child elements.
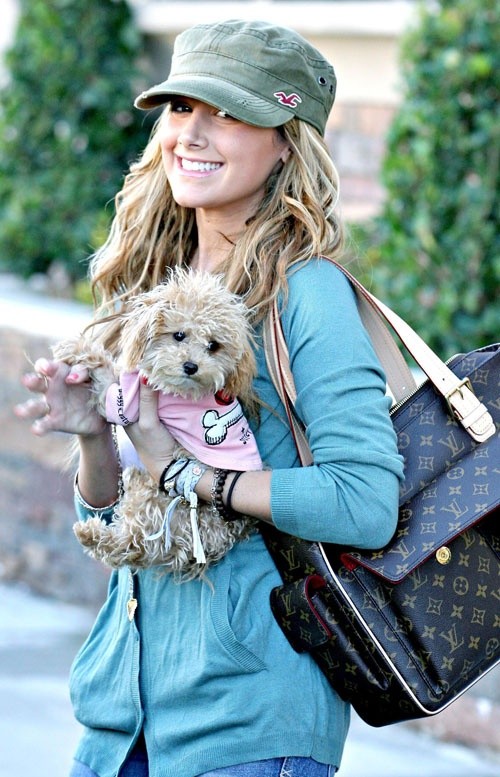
<box><xmin>50</xmin><ymin>267</ymin><xmax>260</xmax><ymax>584</ymax></box>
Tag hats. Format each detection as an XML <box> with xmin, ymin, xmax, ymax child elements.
<box><xmin>133</xmin><ymin>21</ymin><xmax>337</xmax><ymax>136</ymax></box>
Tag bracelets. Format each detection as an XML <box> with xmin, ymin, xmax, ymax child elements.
<box><xmin>158</xmin><ymin>454</ymin><xmax>246</xmax><ymax>566</ymax></box>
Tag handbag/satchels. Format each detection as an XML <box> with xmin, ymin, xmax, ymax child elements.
<box><xmin>262</xmin><ymin>252</ymin><xmax>500</xmax><ymax>727</ymax></box>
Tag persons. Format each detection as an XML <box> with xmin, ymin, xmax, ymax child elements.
<box><xmin>14</xmin><ymin>16</ymin><xmax>407</xmax><ymax>777</ymax></box>
